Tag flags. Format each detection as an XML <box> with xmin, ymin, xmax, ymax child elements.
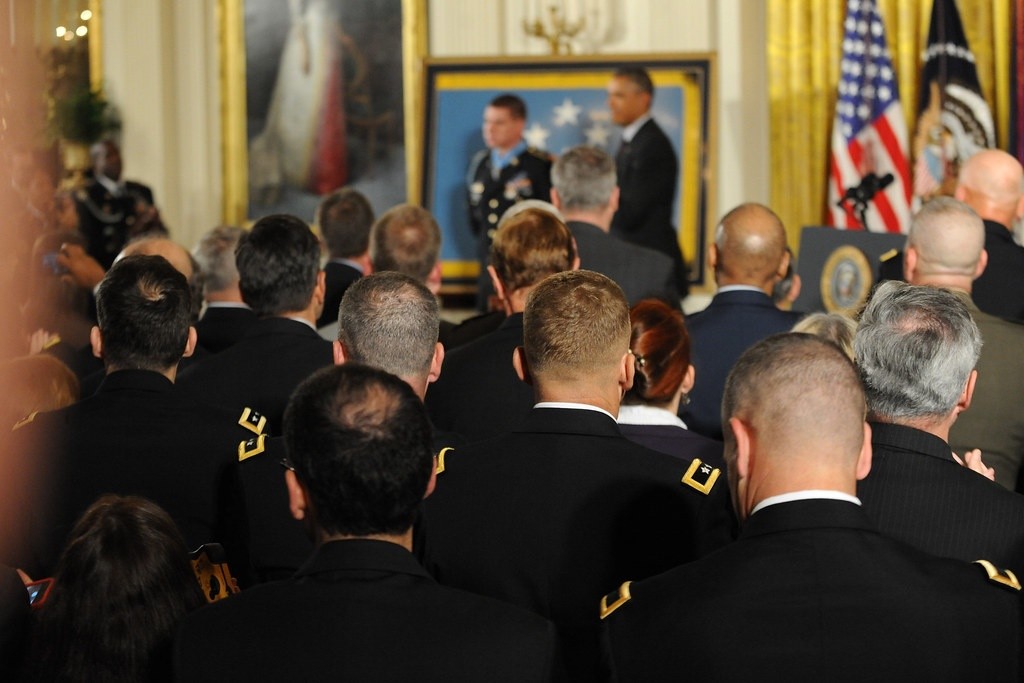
<box><xmin>823</xmin><ymin>0</ymin><xmax>995</xmax><ymax>237</ymax></box>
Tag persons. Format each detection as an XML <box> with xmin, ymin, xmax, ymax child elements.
<box><xmin>0</xmin><ymin>63</ymin><xmax>1024</xmax><ymax>683</ymax></box>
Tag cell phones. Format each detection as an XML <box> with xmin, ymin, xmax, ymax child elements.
<box><xmin>41</xmin><ymin>250</ymin><xmax>71</xmax><ymax>277</ymax></box>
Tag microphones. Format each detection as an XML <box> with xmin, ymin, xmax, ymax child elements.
<box><xmin>859</xmin><ymin>174</ymin><xmax>893</xmax><ymax>200</ymax></box>
